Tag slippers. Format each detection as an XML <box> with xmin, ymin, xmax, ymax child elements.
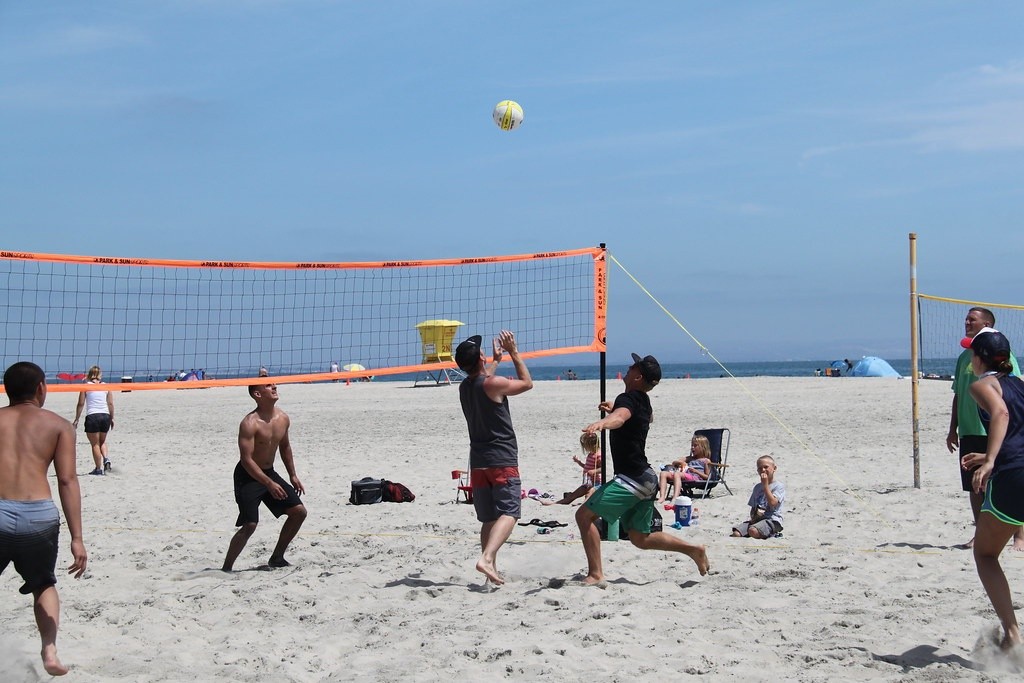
<box><xmin>539</xmin><ymin>493</ymin><xmax>554</xmax><ymax>499</ymax></box>
<box><xmin>541</xmin><ymin>521</ymin><xmax>568</xmax><ymax>527</ymax></box>
<box><xmin>518</xmin><ymin>519</ymin><xmax>544</xmax><ymax>526</ymax></box>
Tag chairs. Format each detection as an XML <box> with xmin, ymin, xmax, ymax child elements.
<box><xmin>451</xmin><ymin>444</ymin><xmax>476</xmax><ymax>505</ymax></box>
<box><xmin>664</xmin><ymin>428</ymin><xmax>734</xmax><ymax>501</ymax></box>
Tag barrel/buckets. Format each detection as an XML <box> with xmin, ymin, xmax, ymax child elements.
<box><xmin>674</xmin><ymin>496</ymin><xmax>692</xmax><ymax>526</ymax></box>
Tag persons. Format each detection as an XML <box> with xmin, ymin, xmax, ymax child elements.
<box><xmin>223</xmin><ymin>375</ymin><xmax>308</xmax><ymax>567</ymax></box>
<box><xmin>455</xmin><ymin>330</ymin><xmax>534</xmax><ymax>586</ymax></box>
<box><xmin>149</xmin><ymin>375</ymin><xmax>153</xmax><ymax>382</ymax></box>
<box><xmin>947</xmin><ymin>306</ymin><xmax>1024</xmax><ymax>552</ymax></box>
<box><xmin>331</xmin><ymin>362</ymin><xmax>339</xmax><ymax>383</ymax></box>
<box><xmin>844</xmin><ymin>359</ymin><xmax>853</xmax><ymax>372</ymax></box>
<box><xmin>0</xmin><ymin>360</ymin><xmax>88</xmax><ymax>676</ymax></box>
<box><xmin>732</xmin><ymin>455</ymin><xmax>784</xmax><ymax>539</ymax></box>
<box><xmin>260</xmin><ymin>365</ymin><xmax>267</xmax><ymax>376</ymax></box>
<box><xmin>174</xmin><ymin>368</ymin><xmax>205</xmax><ymax>380</ymax></box>
<box><xmin>574</xmin><ymin>353</ymin><xmax>710</xmax><ymax>582</ymax></box>
<box><xmin>572</xmin><ymin>433</ymin><xmax>601</xmax><ymax>499</ymax></box>
<box><xmin>567</xmin><ymin>370</ymin><xmax>577</xmax><ymax>380</ymax></box>
<box><xmin>73</xmin><ymin>365</ymin><xmax>115</xmax><ymax>476</ymax></box>
<box><xmin>960</xmin><ymin>326</ymin><xmax>1024</xmax><ymax>650</ymax></box>
<box><xmin>657</xmin><ymin>435</ymin><xmax>712</xmax><ymax>504</ymax></box>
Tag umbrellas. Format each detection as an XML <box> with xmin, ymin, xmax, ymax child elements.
<box><xmin>343</xmin><ymin>364</ymin><xmax>365</xmax><ymax>371</ymax></box>
<box><xmin>831</xmin><ymin>360</ymin><xmax>846</xmax><ymax>367</ymax></box>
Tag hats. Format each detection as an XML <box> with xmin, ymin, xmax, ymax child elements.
<box><xmin>961</xmin><ymin>327</ymin><xmax>1011</xmax><ymax>360</ymax></box>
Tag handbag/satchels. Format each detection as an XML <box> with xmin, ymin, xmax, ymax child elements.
<box><xmin>349</xmin><ymin>477</ymin><xmax>382</xmax><ymax>505</ymax></box>
<box><xmin>601</xmin><ymin>507</ymin><xmax>662</xmax><ymax>540</ymax></box>
<box><xmin>381</xmin><ymin>478</ymin><xmax>415</xmax><ymax>503</ymax></box>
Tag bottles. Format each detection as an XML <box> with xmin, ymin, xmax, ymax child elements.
<box><xmin>537</xmin><ymin>528</ymin><xmax>550</xmax><ymax>534</ymax></box>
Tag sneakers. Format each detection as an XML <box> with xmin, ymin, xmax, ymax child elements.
<box><xmin>103</xmin><ymin>458</ymin><xmax>111</xmax><ymax>472</ymax></box>
<box><xmin>89</xmin><ymin>469</ymin><xmax>103</xmax><ymax>475</ymax></box>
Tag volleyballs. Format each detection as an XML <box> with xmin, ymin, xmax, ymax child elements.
<box><xmin>491</xmin><ymin>99</ymin><xmax>523</xmax><ymax>132</ymax></box>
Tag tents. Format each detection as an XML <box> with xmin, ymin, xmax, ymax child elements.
<box><xmin>847</xmin><ymin>356</ymin><xmax>899</xmax><ymax>376</ymax></box>
